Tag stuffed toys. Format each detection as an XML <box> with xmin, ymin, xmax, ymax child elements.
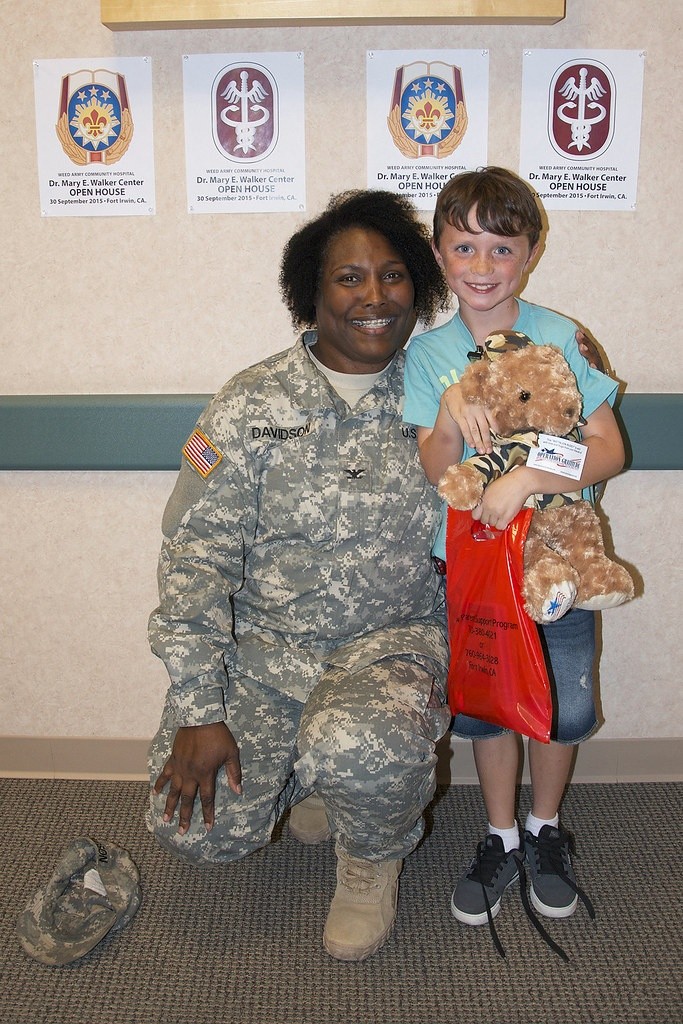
<box><xmin>439</xmin><ymin>331</ymin><xmax>634</xmax><ymax>626</ymax></box>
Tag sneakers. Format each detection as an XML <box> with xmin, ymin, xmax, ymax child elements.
<box><xmin>524</xmin><ymin>820</ymin><xmax>597</xmax><ymax>921</ymax></box>
<box><xmin>289</xmin><ymin>791</ymin><xmax>331</xmax><ymax>844</ymax></box>
<box><xmin>323</xmin><ymin>840</ymin><xmax>402</xmax><ymax>962</ymax></box>
<box><xmin>451</xmin><ymin>834</ymin><xmax>571</xmax><ymax>963</ymax></box>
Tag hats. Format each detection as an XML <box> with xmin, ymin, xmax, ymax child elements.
<box><xmin>17</xmin><ymin>837</ymin><xmax>142</xmax><ymax>967</ymax></box>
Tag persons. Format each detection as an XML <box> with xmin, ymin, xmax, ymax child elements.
<box><xmin>402</xmin><ymin>168</ymin><xmax>625</xmax><ymax>962</ymax></box>
<box><xmin>147</xmin><ymin>192</ymin><xmax>611</xmax><ymax>961</ymax></box>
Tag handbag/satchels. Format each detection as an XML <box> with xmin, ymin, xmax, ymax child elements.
<box><xmin>445</xmin><ymin>504</ymin><xmax>552</xmax><ymax>744</ymax></box>
